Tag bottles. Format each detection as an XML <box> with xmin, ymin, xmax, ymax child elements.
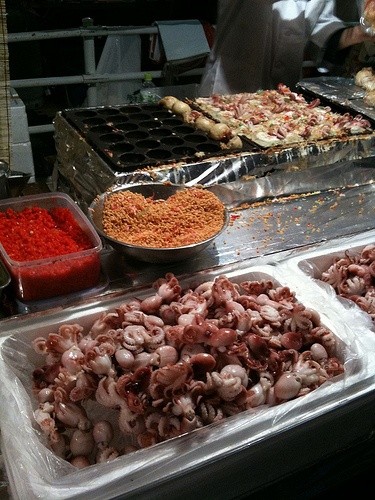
<box><xmin>140</xmin><ymin>71</ymin><xmax>158</xmax><ymax>103</ymax></box>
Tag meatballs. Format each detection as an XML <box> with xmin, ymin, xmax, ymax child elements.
<box><xmin>160</xmin><ymin>96</ymin><xmax>232</xmax><ymax>141</ymax></box>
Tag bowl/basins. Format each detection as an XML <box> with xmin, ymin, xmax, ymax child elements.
<box><xmin>0</xmin><ymin>192</ymin><xmax>104</xmax><ymax>302</ymax></box>
<box><xmin>88</xmin><ymin>181</ymin><xmax>230</xmax><ymax>264</ymax></box>
